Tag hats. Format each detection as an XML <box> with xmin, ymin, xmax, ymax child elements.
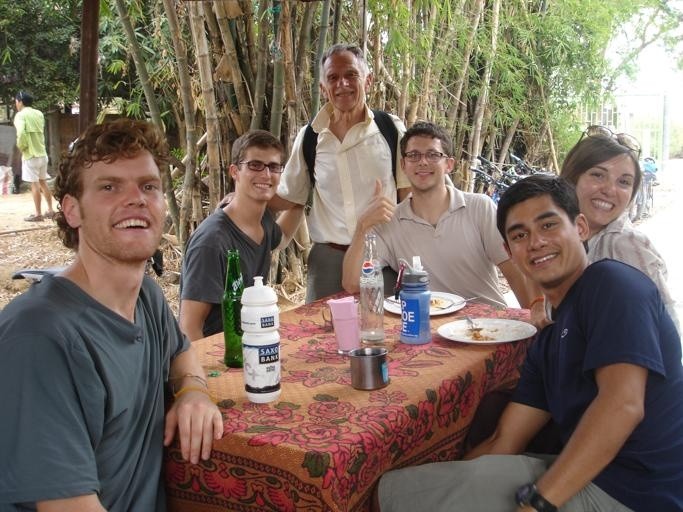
<box><xmin>13</xmin><ymin>91</ymin><xmax>32</xmax><ymax>106</ymax></box>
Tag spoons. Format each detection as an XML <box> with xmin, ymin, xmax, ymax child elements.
<box><xmin>463</xmin><ymin>314</ymin><xmax>483</xmax><ymax>332</ymax></box>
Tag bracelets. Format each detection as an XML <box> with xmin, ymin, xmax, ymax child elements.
<box><xmin>529</xmin><ymin>296</ymin><xmax>544</xmax><ymax>311</ymax></box>
<box><xmin>173</xmin><ymin>386</ymin><xmax>219</xmax><ymax>406</ymax></box>
<box><xmin>170</xmin><ymin>372</ymin><xmax>208</xmax><ymax>388</ymax></box>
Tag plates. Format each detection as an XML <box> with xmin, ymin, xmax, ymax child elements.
<box><xmin>436</xmin><ymin>317</ymin><xmax>538</xmax><ymax>346</ymax></box>
<box><xmin>382</xmin><ymin>291</ymin><xmax>465</xmax><ymax>315</ymax></box>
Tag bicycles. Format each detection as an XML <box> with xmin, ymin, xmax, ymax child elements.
<box><xmin>469</xmin><ymin>152</ymin><xmax>550</xmax><ymax>206</ymax></box>
<box><xmin>626</xmin><ymin>156</ymin><xmax>660</xmax><ymax>226</ymax></box>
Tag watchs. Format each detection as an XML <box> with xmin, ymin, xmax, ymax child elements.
<box><xmin>514</xmin><ymin>482</ymin><xmax>559</xmax><ymax>512</ymax></box>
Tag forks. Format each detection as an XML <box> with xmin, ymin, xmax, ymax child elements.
<box><xmin>435</xmin><ymin>294</ymin><xmax>483</xmax><ymax>310</ymax></box>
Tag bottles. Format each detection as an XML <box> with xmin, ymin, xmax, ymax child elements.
<box><xmin>360</xmin><ymin>234</ymin><xmax>433</xmax><ymax>344</ymax></box>
<box><xmin>220</xmin><ymin>248</ymin><xmax>282</xmax><ymax>404</ymax></box>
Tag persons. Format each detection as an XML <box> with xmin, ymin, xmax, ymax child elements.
<box><xmin>370</xmin><ymin>174</ymin><xmax>683</xmax><ymax>512</ymax></box>
<box><xmin>215</xmin><ymin>44</ymin><xmax>413</xmax><ymax>305</ymax></box>
<box><xmin>13</xmin><ymin>91</ymin><xmax>55</xmax><ymax>222</ymax></box>
<box><xmin>1</xmin><ymin>114</ymin><xmax>224</xmax><ymax>512</ymax></box>
<box><xmin>342</xmin><ymin>122</ymin><xmax>531</xmax><ymax>311</ymax></box>
<box><xmin>175</xmin><ymin>132</ymin><xmax>306</xmax><ymax>346</ymax></box>
<box><xmin>526</xmin><ymin>134</ymin><xmax>682</xmax><ymax>344</ymax></box>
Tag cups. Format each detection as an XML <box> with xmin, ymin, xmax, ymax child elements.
<box><xmin>346</xmin><ymin>347</ymin><xmax>390</xmax><ymax>391</ymax></box>
<box><xmin>321</xmin><ymin>294</ymin><xmax>362</xmax><ymax>352</ymax></box>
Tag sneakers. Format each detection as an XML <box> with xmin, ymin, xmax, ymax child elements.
<box><xmin>22</xmin><ymin>214</ymin><xmax>44</xmax><ymax>222</ymax></box>
<box><xmin>42</xmin><ymin>210</ymin><xmax>56</xmax><ymax>220</ymax></box>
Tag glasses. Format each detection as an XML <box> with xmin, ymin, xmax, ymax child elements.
<box><xmin>401</xmin><ymin>150</ymin><xmax>450</xmax><ymax>163</ymax></box>
<box><xmin>229</xmin><ymin>159</ymin><xmax>286</xmax><ymax>174</ymax></box>
<box><xmin>576</xmin><ymin>124</ymin><xmax>642</xmax><ymax>163</ymax></box>
